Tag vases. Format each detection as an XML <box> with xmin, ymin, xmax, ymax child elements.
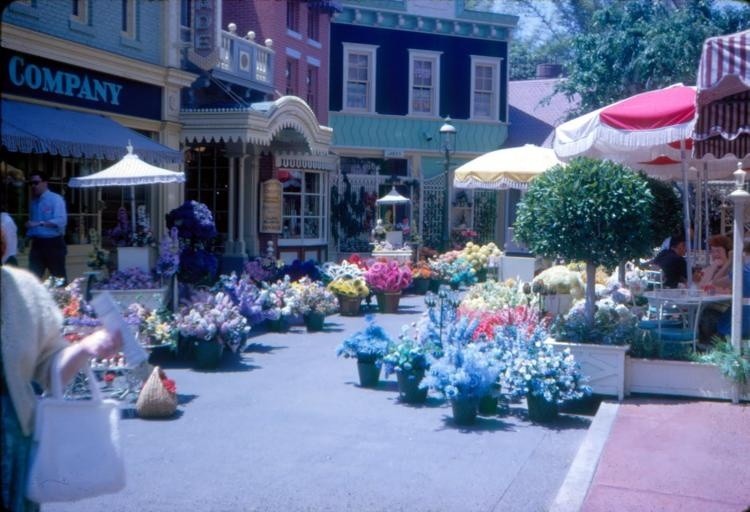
<box><xmin>479</xmin><ymin>393</ymin><xmax>498</xmax><ymax>416</ymax></box>
<box><xmin>262</xmin><ymin>316</ymin><xmax>290</xmax><ymax>332</ymax></box>
<box><xmin>527</xmin><ymin>390</ymin><xmax>559</xmax><ymax>423</ymax></box>
<box><xmin>141</xmin><ymin>344</ymin><xmax>171</xmax><ymax>365</ymax></box>
<box><xmin>302</xmin><ymin>313</ymin><xmax>325</xmax><ymax>332</ymax></box>
<box><xmin>451</xmin><ymin>392</ymin><xmax>475</xmax><ymax>427</ymax></box>
<box><xmin>375</xmin><ymin>291</ymin><xmax>402</xmax><ymax>313</ymax></box>
<box><xmin>356</xmin><ymin>361</ymin><xmax>382</xmax><ymax>387</ymax></box>
<box><xmin>188</xmin><ymin>338</ymin><xmax>222</xmax><ymax>372</ymax></box>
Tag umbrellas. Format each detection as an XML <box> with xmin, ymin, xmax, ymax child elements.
<box><xmin>67</xmin><ymin>139</ymin><xmax>188</xmax><ymax>246</ymax></box>
<box><xmin>376</xmin><ymin>186</ymin><xmax>410</xmax><ymax>230</ymax></box>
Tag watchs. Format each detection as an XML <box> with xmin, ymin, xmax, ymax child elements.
<box><xmin>39</xmin><ymin>221</ymin><xmax>44</xmax><ymax>226</ymax></box>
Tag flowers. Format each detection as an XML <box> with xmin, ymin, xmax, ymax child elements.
<box><xmin>150</xmin><ymin>227</ymin><xmax>184</xmax><ymax>279</ymax></box>
<box><xmin>365</xmin><ymin>261</ymin><xmax>413</xmax><ymax>293</ymax></box>
<box><xmin>172</xmin><ymin>287</ymin><xmax>252</xmax><ymax>354</ymax></box>
<box><xmin>86</xmin><ymin>228</ymin><xmax>115</xmax><ymax>282</ymax></box>
<box><xmin>382</xmin><ymin>275</ymin><xmax>592</xmax><ymax>402</ymax></box>
<box><xmin>209</xmin><ymin>257</ymin><xmax>369</xmax><ymax>324</ymax></box>
<box><xmin>132</xmin><ymin>205</ymin><xmax>156</xmax><ymax>246</ymax></box>
<box><xmin>122</xmin><ymin>301</ymin><xmax>173</xmax><ymax>344</ymax></box>
<box><xmin>396</xmin><ymin>217</ymin><xmax>425</xmax><ymax>244</ymax></box>
<box><xmin>108</xmin><ymin>205</ymin><xmax>131</xmax><ymax>246</ymax></box>
<box><xmin>43</xmin><ymin>275</ymin><xmax>102</xmax><ymax>343</ymax></box>
<box><xmin>162</xmin><ymin>376</ymin><xmax>176</xmax><ymax>396</ymax></box>
<box><xmin>451</xmin><ymin>221</ymin><xmax>480</xmax><ymax>246</ymax></box>
<box><xmin>411</xmin><ymin>241</ymin><xmax>506</xmax><ymax>287</ymax></box>
<box><xmin>91</xmin><ymin>267</ymin><xmax>163</xmax><ymax>289</ymax></box>
<box><xmin>90</xmin><ymin>350</ymin><xmax>126</xmax><ymax>383</ymax></box>
<box><xmin>337</xmin><ymin>314</ymin><xmax>397</xmax><ymax>362</ymax></box>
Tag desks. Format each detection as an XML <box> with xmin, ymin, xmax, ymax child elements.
<box><xmin>640</xmin><ymin>250</ymin><xmax>732</xmax><ymax>356</ymax></box>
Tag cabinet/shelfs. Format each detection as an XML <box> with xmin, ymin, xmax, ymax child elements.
<box><xmin>444</xmin><ymin>167</ymin><xmax>474</xmax><ymax>248</ymax></box>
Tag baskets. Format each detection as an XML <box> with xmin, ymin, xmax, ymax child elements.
<box><xmin>134</xmin><ymin>364</ymin><xmax>178</xmax><ymax>417</ymax></box>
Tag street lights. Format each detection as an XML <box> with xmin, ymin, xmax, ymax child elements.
<box><xmin>438</xmin><ymin>116</ymin><xmax>456</xmax><ymax>254</ymax></box>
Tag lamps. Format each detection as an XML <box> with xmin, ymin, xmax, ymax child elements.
<box><xmin>423</xmin><ymin>128</ymin><xmax>433</xmax><ymax>143</ymax></box>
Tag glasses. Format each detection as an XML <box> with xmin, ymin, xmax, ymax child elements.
<box><xmin>27</xmin><ymin>180</ymin><xmax>44</xmax><ymax>186</ymax></box>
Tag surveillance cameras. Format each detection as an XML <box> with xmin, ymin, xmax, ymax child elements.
<box><xmin>424</xmin><ymin>130</ymin><xmax>432</xmax><ymax>141</ymax></box>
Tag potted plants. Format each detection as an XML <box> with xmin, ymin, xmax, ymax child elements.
<box><xmin>626</xmin><ymin>327</ymin><xmax>750</xmax><ymax>404</ymax></box>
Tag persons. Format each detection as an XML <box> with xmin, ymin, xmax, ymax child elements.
<box><xmin>0</xmin><ymin>211</ymin><xmax>18</xmax><ymax>265</ymax></box>
<box><xmin>0</xmin><ymin>227</ymin><xmax>123</xmax><ymax>512</ymax></box>
<box><xmin>25</xmin><ymin>171</ymin><xmax>70</xmax><ymax>286</ymax></box>
<box><xmin>652</xmin><ymin>231</ymin><xmax>750</xmax><ymax>340</ymax></box>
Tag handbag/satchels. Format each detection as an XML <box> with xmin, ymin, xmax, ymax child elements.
<box><xmin>25</xmin><ymin>347</ymin><xmax>126</xmax><ymax>504</ymax></box>
